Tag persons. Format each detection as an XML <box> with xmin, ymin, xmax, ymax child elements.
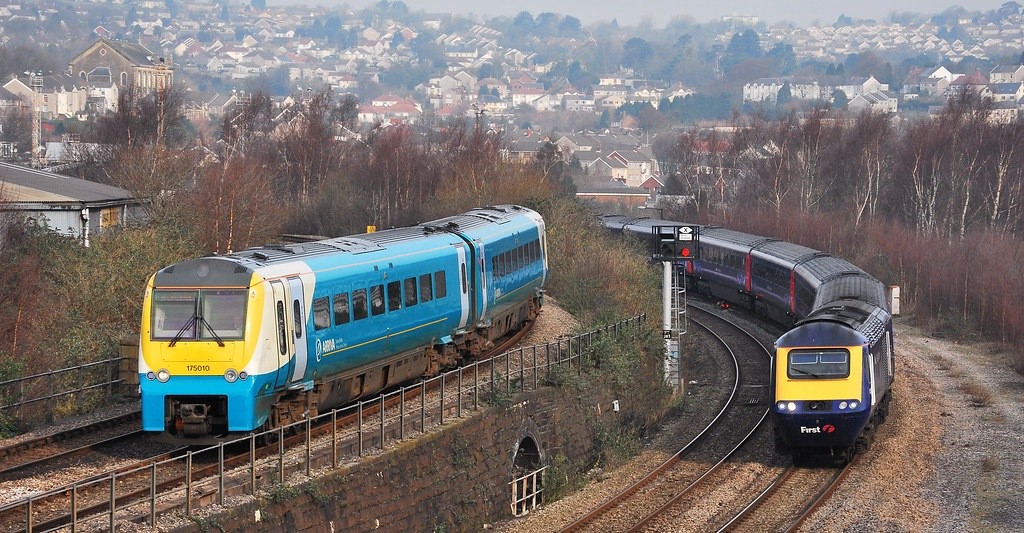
<box><xmin>390</xmin><ymin>295</ymin><xmax>399</xmax><ymax>310</ymax></box>
<box><xmin>357</xmin><ymin>304</ymin><xmax>367</xmax><ymax>318</ymax></box>
<box><xmin>422</xmin><ymin>289</ymin><xmax>429</xmax><ymax>301</ymax></box>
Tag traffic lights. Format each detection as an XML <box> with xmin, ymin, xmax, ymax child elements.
<box><xmin>659</xmin><ymin>242</ymin><xmax>696</xmax><ymax>259</ymax></box>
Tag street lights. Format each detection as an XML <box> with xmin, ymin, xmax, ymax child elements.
<box><xmin>637</xmin><ymin>206</ymin><xmax>663</xmax><ymax>219</ymax></box>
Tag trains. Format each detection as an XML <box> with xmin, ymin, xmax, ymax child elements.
<box><xmin>136</xmin><ymin>201</ymin><xmax>549</xmax><ymax>442</ymax></box>
<box><xmin>596</xmin><ymin>213</ymin><xmax>895</xmax><ymax>465</ymax></box>
